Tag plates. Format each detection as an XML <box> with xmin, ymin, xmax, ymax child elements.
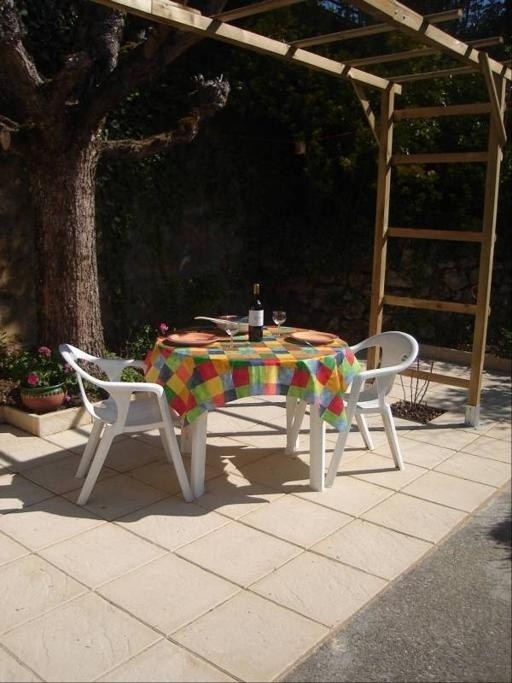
<box><xmin>169</xmin><ymin>333</ymin><xmax>219</xmax><ymax>347</ymax></box>
<box><xmin>290</xmin><ymin>332</ymin><xmax>335</xmax><ymax>346</ymax></box>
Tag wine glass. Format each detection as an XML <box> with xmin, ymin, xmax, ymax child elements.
<box><xmin>225</xmin><ymin>315</ymin><xmax>239</xmax><ymax>350</ymax></box>
<box><xmin>272</xmin><ymin>309</ymin><xmax>286</xmax><ymax>337</ymax></box>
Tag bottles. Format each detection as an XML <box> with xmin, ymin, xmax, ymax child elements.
<box><xmin>249</xmin><ymin>283</ymin><xmax>264</xmax><ymax>343</ymax></box>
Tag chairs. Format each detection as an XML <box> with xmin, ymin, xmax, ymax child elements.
<box><xmin>57</xmin><ymin>341</ymin><xmax>194</xmax><ymax>507</ymax></box>
<box><xmin>284</xmin><ymin>328</ymin><xmax>419</xmax><ymax>492</ymax></box>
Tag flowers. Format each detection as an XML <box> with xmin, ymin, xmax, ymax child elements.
<box><xmin>8</xmin><ymin>340</ymin><xmax>77</xmax><ymax>403</ymax></box>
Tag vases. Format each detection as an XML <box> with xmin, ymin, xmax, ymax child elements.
<box><xmin>21</xmin><ymin>381</ymin><xmax>69</xmax><ymax>415</ymax></box>
